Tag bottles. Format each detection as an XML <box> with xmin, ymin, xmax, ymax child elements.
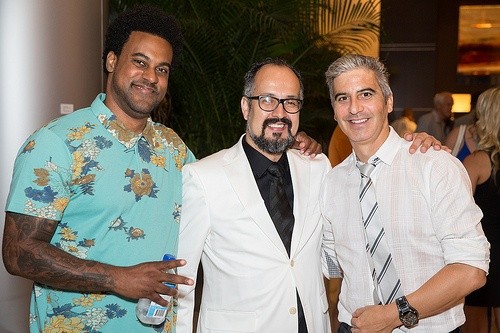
<box><xmin>136</xmin><ymin>254</ymin><xmax>178</xmax><ymax>326</ymax></box>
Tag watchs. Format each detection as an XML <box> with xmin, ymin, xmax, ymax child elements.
<box><xmin>395</xmin><ymin>295</ymin><xmax>420</xmax><ymax>328</ymax></box>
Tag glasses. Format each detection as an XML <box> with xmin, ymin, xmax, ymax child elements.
<box><xmin>248</xmin><ymin>94</ymin><xmax>303</xmax><ymax>115</ymax></box>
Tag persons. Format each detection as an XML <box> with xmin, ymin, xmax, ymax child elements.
<box><xmin>325</xmin><ymin>53</ymin><xmax>491</xmax><ymax>332</ymax></box>
<box><xmin>419</xmin><ymin>92</ymin><xmax>479</xmax><ymax>161</ymax></box>
<box><xmin>391</xmin><ymin>108</ymin><xmax>417</xmax><ymax>138</ymax></box>
<box><xmin>2</xmin><ymin>17</ymin><xmax>322</xmax><ymax>332</ymax></box>
<box><xmin>462</xmin><ymin>88</ymin><xmax>500</xmax><ymax>333</ymax></box>
<box><xmin>177</xmin><ymin>57</ymin><xmax>332</xmax><ymax>333</ymax></box>
<box><xmin>327</xmin><ymin>123</ymin><xmax>352</xmax><ymax>167</ymax></box>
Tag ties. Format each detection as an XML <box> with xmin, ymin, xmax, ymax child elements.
<box><xmin>355</xmin><ymin>157</ymin><xmax>405</xmax><ymax>305</ymax></box>
<box><xmin>267</xmin><ymin>163</ymin><xmax>295</xmax><ymax>256</ymax></box>
